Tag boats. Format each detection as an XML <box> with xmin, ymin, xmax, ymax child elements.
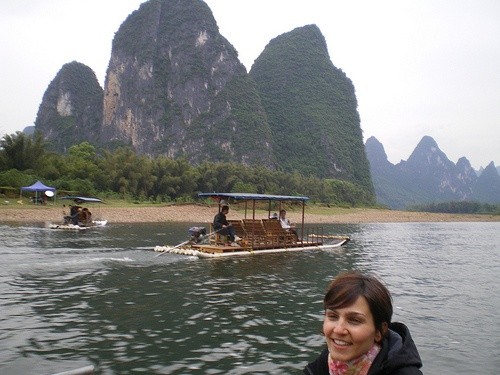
<box><xmin>50</xmin><ymin>196</ymin><xmax>108</xmax><ymax>230</ymax></box>
<box><xmin>155</xmin><ymin>193</ymin><xmax>350</xmax><ymax>258</ymax></box>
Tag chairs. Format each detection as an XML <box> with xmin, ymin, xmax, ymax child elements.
<box><xmin>209</xmin><ymin>219</ymin><xmax>298</xmax><ymax>247</ymax></box>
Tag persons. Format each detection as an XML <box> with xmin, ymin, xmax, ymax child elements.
<box><xmin>213</xmin><ymin>206</ymin><xmax>243</xmax><ymax>247</ymax></box>
<box><xmin>302</xmin><ymin>273</ymin><xmax>425</xmax><ymax>375</ymax></box>
<box><xmin>279</xmin><ymin>209</ymin><xmax>300</xmax><ymax>241</ymax></box>
<box><xmin>68</xmin><ymin>204</ymin><xmax>82</xmax><ymax>225</ymax></box>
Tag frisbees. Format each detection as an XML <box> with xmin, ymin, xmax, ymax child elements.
<box><xmin>45</xmin><ymin>191</ymin><xmax>54</xmax><ymax>197</ymax></box>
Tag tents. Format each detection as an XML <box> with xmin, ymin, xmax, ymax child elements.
<box><xmin>20</xmin><ymin>180</ymin><xmax>57</xmax><ymax>205</ymax></box>
